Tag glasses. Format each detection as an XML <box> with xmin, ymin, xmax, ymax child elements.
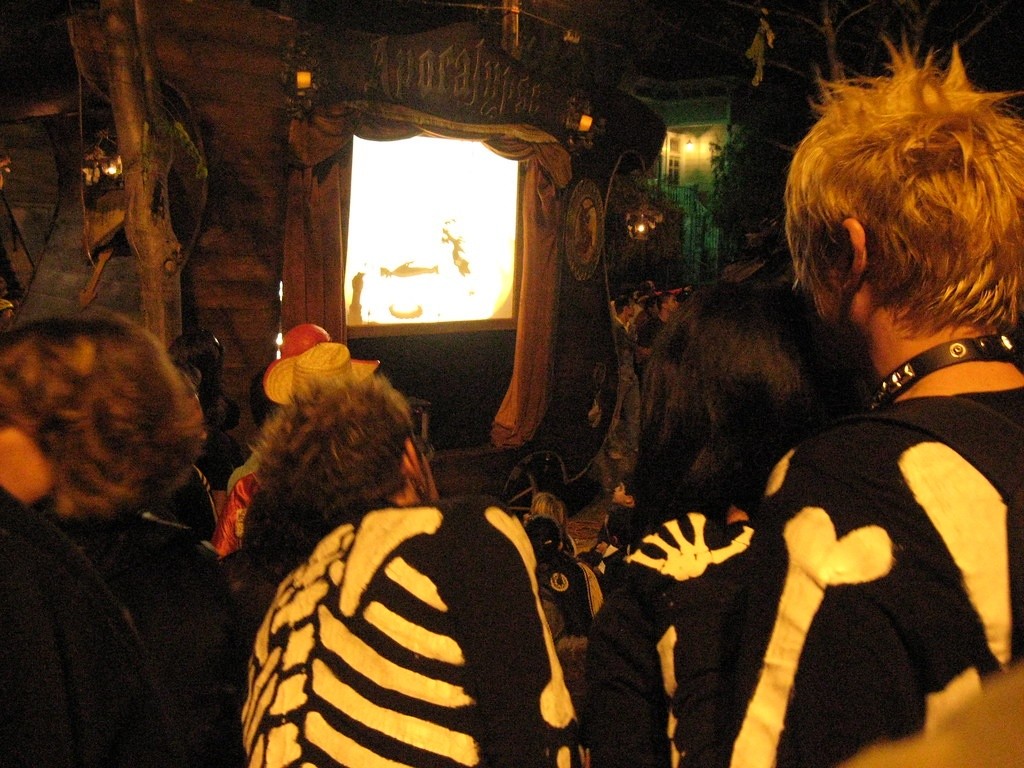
<box><xmin>196</xmin><ymin>326</ymin><xmax>221</xmax><ymax>349</ymax></box>
<box><xmin>667</xmin><ymin>291</ymin><xmax>688</xmax><ymax>302</ymax></box>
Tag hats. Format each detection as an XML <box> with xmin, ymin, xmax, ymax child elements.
<box><xmin>263</xmin><ymin>324</ymin><xmax>380</xmax><ymax>387</ymax></box>
<box><xmin>265</xmin><ymin>342</ymin><xmax>377</xmax><ymax>404</ymax></box>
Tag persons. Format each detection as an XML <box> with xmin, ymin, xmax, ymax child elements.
<box><xmin>622</xmin><ymin>29</ymin><xmax>1024</xmax><ymax>768</ymax></box>
<box><xmin>0</xmin><ymin>278</ymin><xmax>870</xmax><ymax>767</ymax></box>
<box><xmin>240</xmin><ymin>370</ymin><xmax>591</xmax><ymax>768</ymax></box>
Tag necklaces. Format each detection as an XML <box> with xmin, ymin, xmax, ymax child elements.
<box><xmin>862</xmin><ymin>333</ymin><xmax>1014</xmax><ymax>409</ymax></box>
